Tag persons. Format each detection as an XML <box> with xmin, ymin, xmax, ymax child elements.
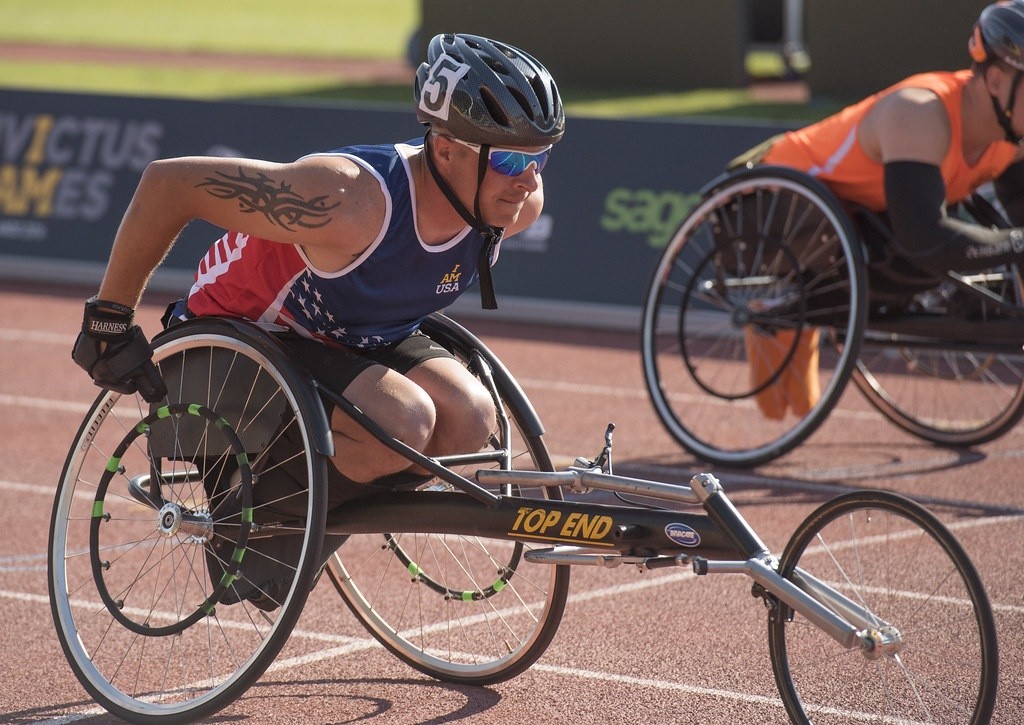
<box><xmin>70</xmin><ymin>33</ymin><xmax>565</xmax><ymax>607</ymax></box>
<box><xmin>746</xmin><ymin>0</ymin><xmax>1024</xmax><ymax>420</ymax></box>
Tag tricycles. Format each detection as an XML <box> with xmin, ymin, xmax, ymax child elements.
<box><xmin>639</xmin><ymin>155</ymin><xmax>1024</xmax><ymax>471</ymax></box>
<box><xmin>48</xmin><ymin>294</ymin><xmax>999</xmax><ymax>725</ymax></box>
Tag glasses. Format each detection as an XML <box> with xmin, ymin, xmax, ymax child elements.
<box><xmin>429</xmin><ymin>131</ymin><xmax>554</xmax><ymax>176</ymax></box>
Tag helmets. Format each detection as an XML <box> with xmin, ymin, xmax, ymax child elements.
<box><xmin>412</xmin><ymin>31</ymin><xmax>567</xmax><ymax>150</ymax></box>
<box><xmin>977</xmin><ymin>2</ymin><xmax>1024</xmax><ymax>72</ymax></box>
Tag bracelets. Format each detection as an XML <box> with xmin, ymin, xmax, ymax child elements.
<box><xmin>95</xmin><ymin>300</ymin><xmax>132</xmax><ymax>314</ymax></box>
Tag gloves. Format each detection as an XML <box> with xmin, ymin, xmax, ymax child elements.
<box><xmin>67</xmin><ymin>299</ymin><xmax>169</xmax><ymax>404</ymax></box>
